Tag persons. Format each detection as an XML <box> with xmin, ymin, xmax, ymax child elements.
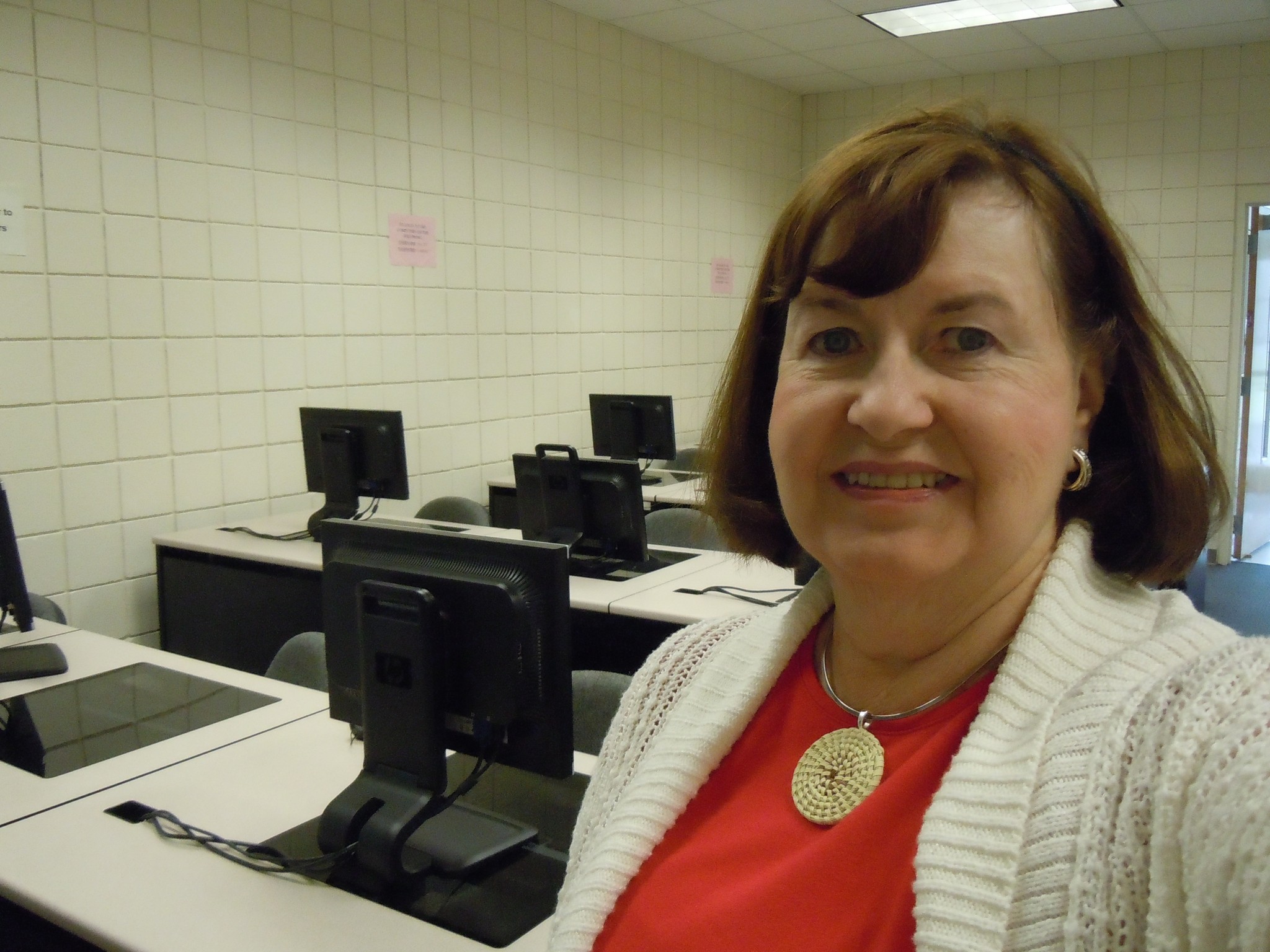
<box><xmin>549</xmin><ymin>101</ymin><xmax>1270</xmax><ymax>952</ymax></box>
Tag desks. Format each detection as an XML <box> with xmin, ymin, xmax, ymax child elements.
<box><xmin>0</xmin><ymin>469</ymin><xmax>798</xmax><ymax>952</ymax></box>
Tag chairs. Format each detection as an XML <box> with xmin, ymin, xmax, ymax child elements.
<box><xmin>570</xmin><ymin>670</ymin><xmax>632</xmax><ymax>756</ymax></box>
<box><xmin>263</xmin><ymin>631</ymin><xmax>327</xmax><ymax>692</ymax></box>
<box><xmin>644</xmin><ymin>506</ymin><xmax>733</xmax><ymax>554</ymax></box>
<box><xmin>414</xmin><ymin>497</ymin><xmax>491</xmax><ymax>528</ymax></box>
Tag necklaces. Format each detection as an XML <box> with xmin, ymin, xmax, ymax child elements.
<box><xmin>791</xmin><ymin>625</ymin><xmax>1015</xmax><ymax>825</ymax></box>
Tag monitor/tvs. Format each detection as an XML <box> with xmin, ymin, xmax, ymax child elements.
<box><xmin>515</xmin><ymin>443</ymin><xmax>648</xmax><ymax>576</ymax></box>
<box><xmin>317</xmin><ymin>517</ymin><xmax>573</xmax><ymax>888</ymax></box>
<box><xmin>300</xmin><ymin>407</ymin><xmax>409</xmax><ymax>539</ymax></box>
<box><xmin>590</xmin><ymin>393</ymin><xmax>675</xmax><ymax>484</ymax></box>
